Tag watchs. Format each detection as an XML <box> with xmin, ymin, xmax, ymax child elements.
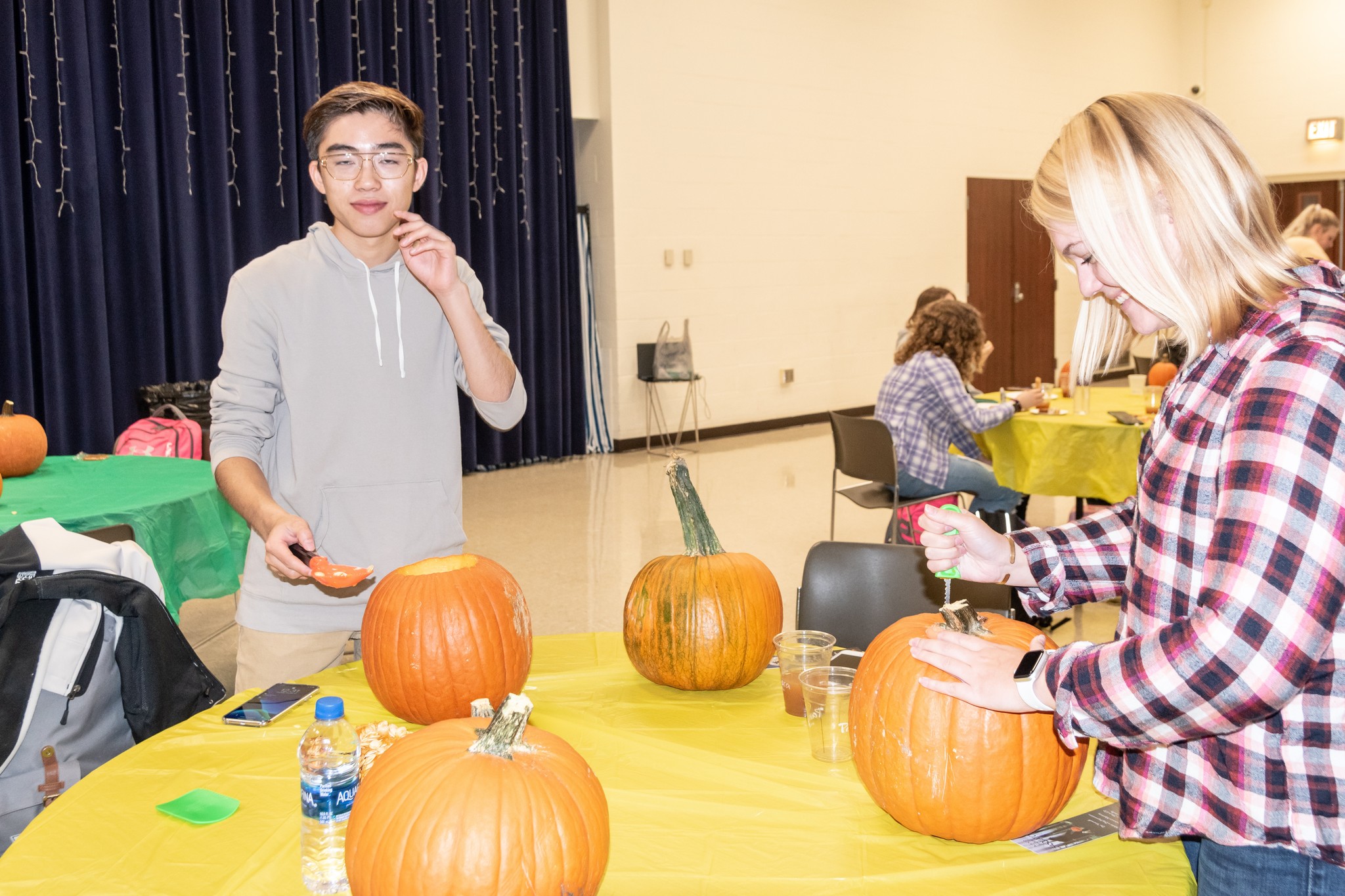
<box><xmin>1012</xmin><ymin>649</ymin><xmax>1057</xmax><ymax>712</ymax></box>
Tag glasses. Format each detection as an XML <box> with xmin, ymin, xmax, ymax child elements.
<box><xmin>318</xmin><ymin>152</ymin><xmax>416</xmax><ymax>180</ymax></box>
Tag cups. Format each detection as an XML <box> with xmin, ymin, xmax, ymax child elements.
<box><xmin>1073</xmin><ymin>384</ymin><xmax>1090</xmax><ymax>416</ymax></box>
<box><xmin>773</xmin><ymin>630</ymin><xmax>836</xmax><ymax>717</ymax></box>
<box><xmin>1143</xmin><ymin>385</ymin><xmax>1164</xmax><ymax>414</ymax></box>
<box><xmin>1031</xmin><ymin>382</ymin><xmax>1053</xmax><ymax>409</ymax></box>
<box><xmin>799</xmin><ymin>665</ymin><xmax>858</xmax><ymax>761</ymax></box>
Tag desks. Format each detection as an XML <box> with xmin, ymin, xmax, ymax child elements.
<box><xmin>962</xmin><ymin>382</ymin><xmax>1165</xmax><ymax>517</ymax></box>
<box><xmin>0</xmin><ymin>618</ymin><xmax>1195</xmax><ymax>896</ymax></box>
<box><xmin>0</xmin><ymin>450</ymin><xmax>250</xmax><ymax>624</ymax></box>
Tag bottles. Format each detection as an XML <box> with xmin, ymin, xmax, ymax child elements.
<box><xmin>298</xmin><ymin>697</ymin><xmax>361</xmax><ymax>896</ymax></box>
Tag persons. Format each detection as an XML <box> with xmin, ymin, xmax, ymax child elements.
<box><xmin>1276</xmin><ymin>198</ymin><xmax>1344</xmax><ymax>273</ymax></box>
<box><xmin>870</xmin><ymin>298</ymin><xmax>1046</xmax><ymax>526</ymax></box>
<box><xmin>205</xmin><ymin>78</ymin><xmax>530</xmax><ymax>696</ymax></box>
<box><xmin>908</xmin><ymin>91</ymin><xmax>1345</xmax><ymax>895</ymax></box>
<box><xmin>896</xmin><ymin>288</ymin><xmax>959</xmax><ymax>359</ymax></box>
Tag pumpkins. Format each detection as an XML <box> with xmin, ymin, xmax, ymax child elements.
<box><xmin>361</xmin><ymin>554</ymin><xmax>533</xmax><ymax>725</ymax></box>
<box><xmin>1147</xmin><ymin>352</ymin><xmax>1177</xmax><ymax>385</ymax></box>
<box><xmin>622</xmin><ymin>457</ymin><xmax>784</xmax><ymax>690</ymax></box>
<box><xmin>848</xmin><ymin>599</ymin><xmax>1090</xmax><ymax>844</ymax></box>
<box><xmin>345</xmin><ymin>692</ymin><xmax>610</xmax><ymax>896</ymax></box>
<box><xmin>0</xmin><ymin>399</ymin><xmax>48</xmax><ymax>496</ymax></box>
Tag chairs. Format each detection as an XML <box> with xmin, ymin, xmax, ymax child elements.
<box><xmin>793</xmin><ymin>537</ymin><xmax>953</xmax><ymax>653</ymax></box>
<box><xmin>825</xmin><ymin>411</ymin><xmax>968</xmax><ymax>547</ymax></box>
<box><xmin>637</xmin><ymin>340</ymin><xmax>704</xmax><ymax>461</ymax></box>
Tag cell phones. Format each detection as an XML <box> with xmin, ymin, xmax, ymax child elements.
<box><xmin>222</xmin><ymin>682</ymin><xmax>321</xmax><ymax>727</ymax></box>
<box><xmin>1107</xmin><ymin>410</ymin><xmax>1144</xmax><ymax>426</ymax></box>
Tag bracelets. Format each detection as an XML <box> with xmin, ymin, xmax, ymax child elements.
<box><xmin>998</xmin><ymin>533</ymin><xmax>1016</xmax><ymax>585</ymax></box>
<box><xmin>1008</xmin><ymin>398</ymin><xmax>1022</xmax><ymax>412</ymax></box>
